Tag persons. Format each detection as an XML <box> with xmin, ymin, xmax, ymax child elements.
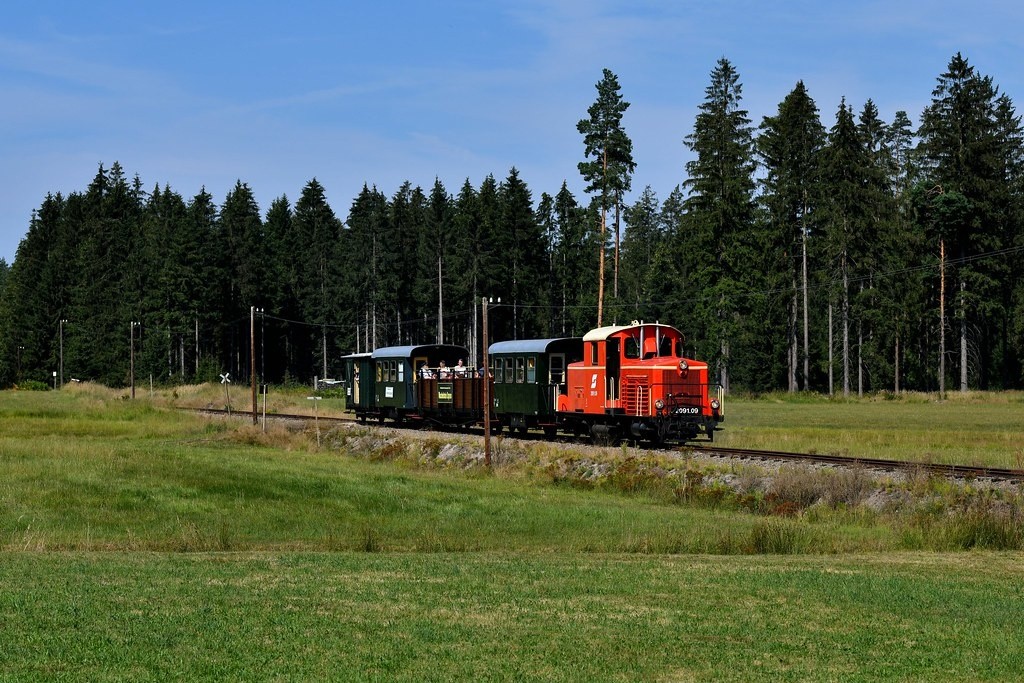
<box><xmin>417</xmin><ymin>359</ymin><xmax>494</xmax><ymax>379</ymax></box>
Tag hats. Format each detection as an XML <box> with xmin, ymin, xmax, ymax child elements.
<box><xmin>416</xmin><ymin>360</ymin><xmax>426</xmax><ymax>370</ymax></box>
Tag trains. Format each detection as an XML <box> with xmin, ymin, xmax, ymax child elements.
<box><xmin>345</xmin><ymin>319</ymin><xmax>725</xmax><ymax>449</ymax></box>
